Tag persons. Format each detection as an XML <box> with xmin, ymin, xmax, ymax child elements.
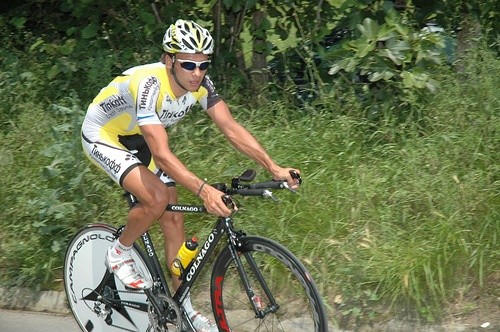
<box><xmin>80</xmin><ymin>19</ymin><xmax>299</xmax><ymax>332</ymax></box>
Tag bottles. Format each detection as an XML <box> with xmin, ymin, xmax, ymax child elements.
<box><xmin>170</xmin><ymin>237</ymin><xmax>198</xmax><ymax>277</ymax></box>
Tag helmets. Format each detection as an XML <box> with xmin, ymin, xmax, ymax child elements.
<box><xmin>163</xmin><ymin>19</ymin><xmax>214</xmax><ymax>56</ymax></box>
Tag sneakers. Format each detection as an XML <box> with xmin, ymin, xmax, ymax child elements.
<box><xmin>104</xmin><ymin>245</ymin><xmax>153</xmax><ymax>290</ymax></box>
<box><xmin>181</xmin><ymin>311</ymin><xmax>232</xmax><ymax>332</ymax></box>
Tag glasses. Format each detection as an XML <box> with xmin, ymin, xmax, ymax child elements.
<box><xmin>176</xmin><ymin>59</ymin><xmax>211</xmax><ymax>70</ymax></box>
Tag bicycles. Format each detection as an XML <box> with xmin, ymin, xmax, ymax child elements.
<box><xmin>62</xmin><ymin>150</ymin><xmax>328</xmax><ymax>332</ymax></box>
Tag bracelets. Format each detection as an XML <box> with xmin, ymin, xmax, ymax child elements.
<box><xmin>197</xmin><ymin>181</ymin><xmax>207</xmax><ymax>197</ymax></box>
